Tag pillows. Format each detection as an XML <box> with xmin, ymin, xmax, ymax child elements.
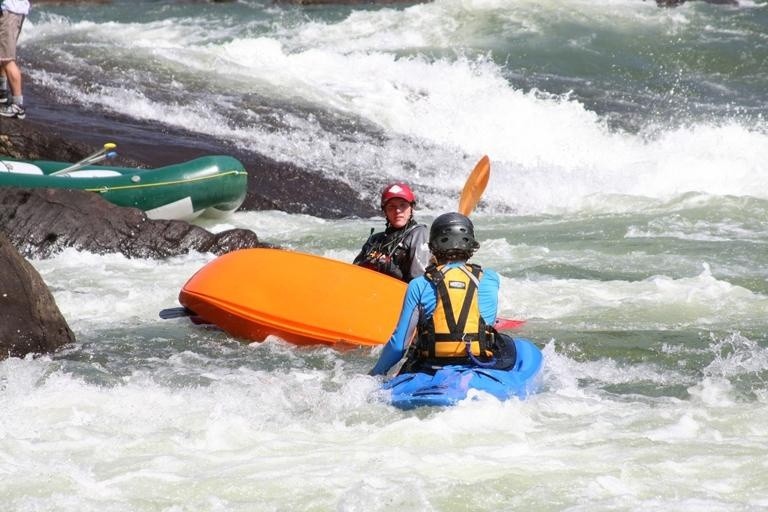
<box><xmin>428</xmin><ymin>212</ymin><xmax>480</xmax><ymax>260</ymax></box>
<box><xmin>380</xmin><ymin>182</ymin><xmax>416</xmax><ymax>209</ymax></box>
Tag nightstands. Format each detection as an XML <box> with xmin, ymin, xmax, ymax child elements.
<box><xmin>460</xmin><ymin>155</ymin><xmax>490</xmax><ymax>216</ymax></box>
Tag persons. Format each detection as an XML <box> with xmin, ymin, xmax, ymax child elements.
<box><xmin>366</xmin><ymin>212</ymin><xmax>501</xmax><ymax>377</ymax></box>
<box><xmin>0</xmin><ymin>0</ymin><xmax>30</xmax><ymax>121</ymax></box>
<box><xmin>352</xmin><ymin>182</ymin><xmax>432</xmax><ymax>284</ymax></box>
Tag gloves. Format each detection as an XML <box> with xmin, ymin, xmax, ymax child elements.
<box><xmin>0</xmin><ymin>151</ymin><xmax>249</xmax><ymax>221</ymax></box>
<box><xmin>178</xmin><ymin>248</ymin><xmax>527</xmax><ymax>354</ymax></box>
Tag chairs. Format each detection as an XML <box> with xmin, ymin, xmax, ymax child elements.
<box><xmin>0</xmin><ymin>88</ymin><xmax>8</xmax><ymax>104</ymax></box>
<box><xmin>0</xmin><ymin>103</ymin><xmax>26</xmax><ymax>120</ymax></box>
